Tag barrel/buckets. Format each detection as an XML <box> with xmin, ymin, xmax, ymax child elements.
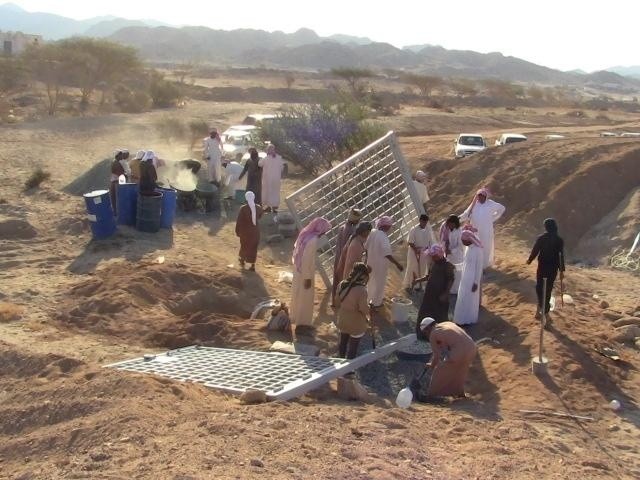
<box><xmin>196</xmin><ymin>182</ymin><xmax>218</xmax><ymax>215</ymax></box>
<box><xmin>275</xmin><ymin>208</ymin><xmax>297</xmax><ymax>235</ymax></box>
<box><xmin>235</xmin><ymin>189</ymin><xmax>247</xmax><ymax>203</ymax></box>
<box><xmin>395</xmin><ymin>384</ymin><xmax>413</xmax><ymax>409</ymax></box>
<box><xmin>391</xmin><ymin>296</ymin><xmax>413</xmax><ymax>324</ymax></box>
<box><xmin>83</xmin><ymin>183</ymin><xmax>177</xmax><ymax>239</ymax></box>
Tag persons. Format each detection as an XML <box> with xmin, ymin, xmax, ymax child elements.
<box><xmin>459</xmin><ymin>189</ymin><xmax>506</xmax><ymax>270</ymax></box>
<box><xmin>527</xmin><ymin>218</ymin><xmax>565</xmax><ymax>320</ymax></box>
<box><xmin>290</xmin><ymin>217</ymin><xmax>332</xmax><ymax>334</ymax></box>
<box><xmin>201</xmin><ymin>127</ymin><xmax>223</xmax><ymax>189</ymax></box>
<box><xmin>235</xmin><ymin>191</ymin><xmax>264</xmax><ymax>272</ymax></box>
<box><xmin>334</xmin><ymin>261</ymin><xmax>372</xmax><ymax>374</ymax></box>
<box><xmin>110</xmin><ymin>145</ymin><xmax>284</xmax><ymax>216</ymax></box>
<box><xmin>396</xmin><ymin>170</ymin><xmax>429</xmax><ymax>244</ymax></box>
<box><xmin>420</xmin><ymin>317</ymin><xmax>477</xmax><ymax>400</ymax></box>
<box><xmin>333</xmin><ymin>208</ymin><xmax>484</xmax><ymax>341</ymax></box>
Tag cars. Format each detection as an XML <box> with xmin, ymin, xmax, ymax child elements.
<box><xmin>543</xmin><ymin>134</ymin><xmax>566</xmax><ymax>140</ymax></box>
<box><xmin>622</xmin><ymin>132</ymin><xmax>639</xmax><ymax>136</ymax></box>
<box><xmin>201</xmin><ymin>111</ymin><xmax>324</xmax><ymax>178</ymax></box>
<box><xmin>599</xmin><ymin>132</ymin><xmax>617</xmax><ymax>137</ymax></box>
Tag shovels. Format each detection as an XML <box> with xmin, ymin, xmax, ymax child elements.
<box><xmin>409</xmin><ymin>358</ymin><xmax>433</xmax><ymax>396</ymax></box>
<box><xmin>414</xmin><ymin>250</ymin><xmax>424</xmax><ymax>292</ymax></box>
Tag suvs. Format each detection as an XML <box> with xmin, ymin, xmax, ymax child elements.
<box><xmin>454</xmin><ymin>133</ymin><xmax>487</xmax><ymax>158</ymax></box>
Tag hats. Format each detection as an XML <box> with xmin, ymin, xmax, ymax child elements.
<box><xmin>420</xmin><ymin>317</ymin><xmax>435</xmax><ymax>330</ymax></box>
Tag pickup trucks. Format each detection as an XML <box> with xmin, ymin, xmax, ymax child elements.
<box><xmin>494</xmin><ymin>133</ymin><xmax>527</xmax><ymax>147</ymax></box>
